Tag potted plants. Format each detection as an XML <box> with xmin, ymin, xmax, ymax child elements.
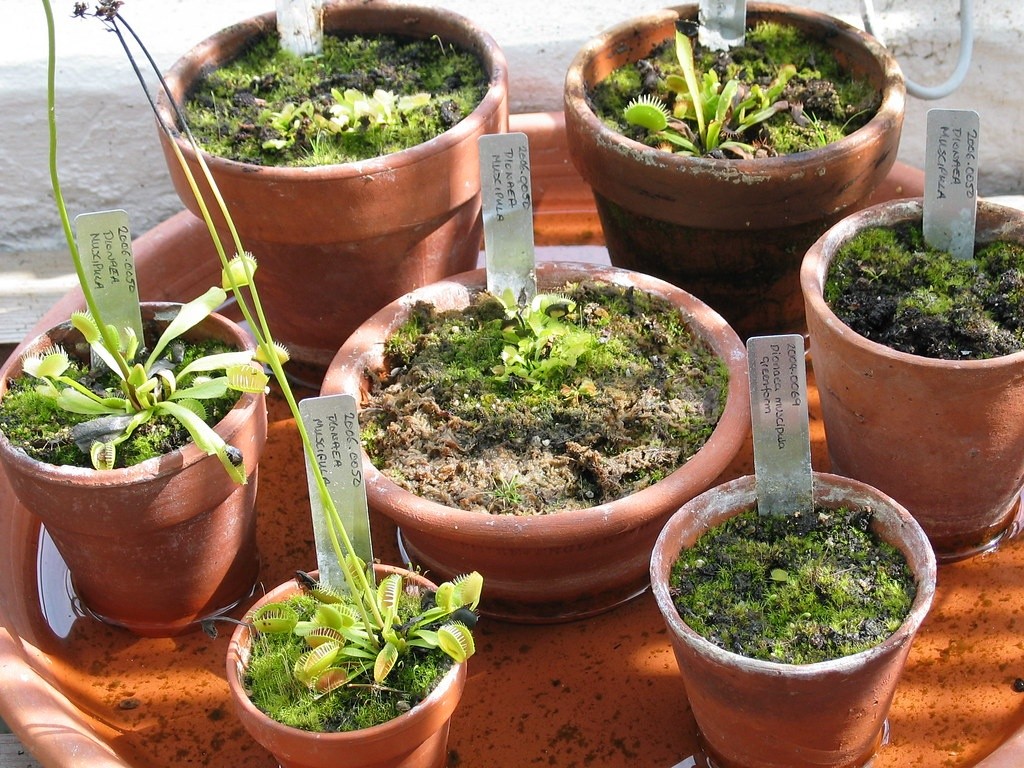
<box><xmin>800</xmin><ymin>195</ymin><xmax>1024</xmax><ymax>563</ymax></box>
<box><xmin>69</xmin><ymin>0</ymin><xmax>482</xmax><ymax>767</ymax></box>
<box><xmin>563</xmin><ymin>1</ymin><xmax>907</xmax><ymax>348</ymax></box>
<box><xmin>320</xmin><ymin>260</ymin><xmax>753</xmax><ymax>625</ymax></box>
<box><xmin>156</xmin><ymin>0</ymin><xmax>510</xmax><ymax>389</ymax></box>
<box><xmin>0</xmin><ymin>0</ymin><xmax>268</xmax><ymax>634</ymax></box>
<box><xmin>649</xmin><ymin>469</ymin><xmax>937</xmax><ymax>768</ymax></box>
